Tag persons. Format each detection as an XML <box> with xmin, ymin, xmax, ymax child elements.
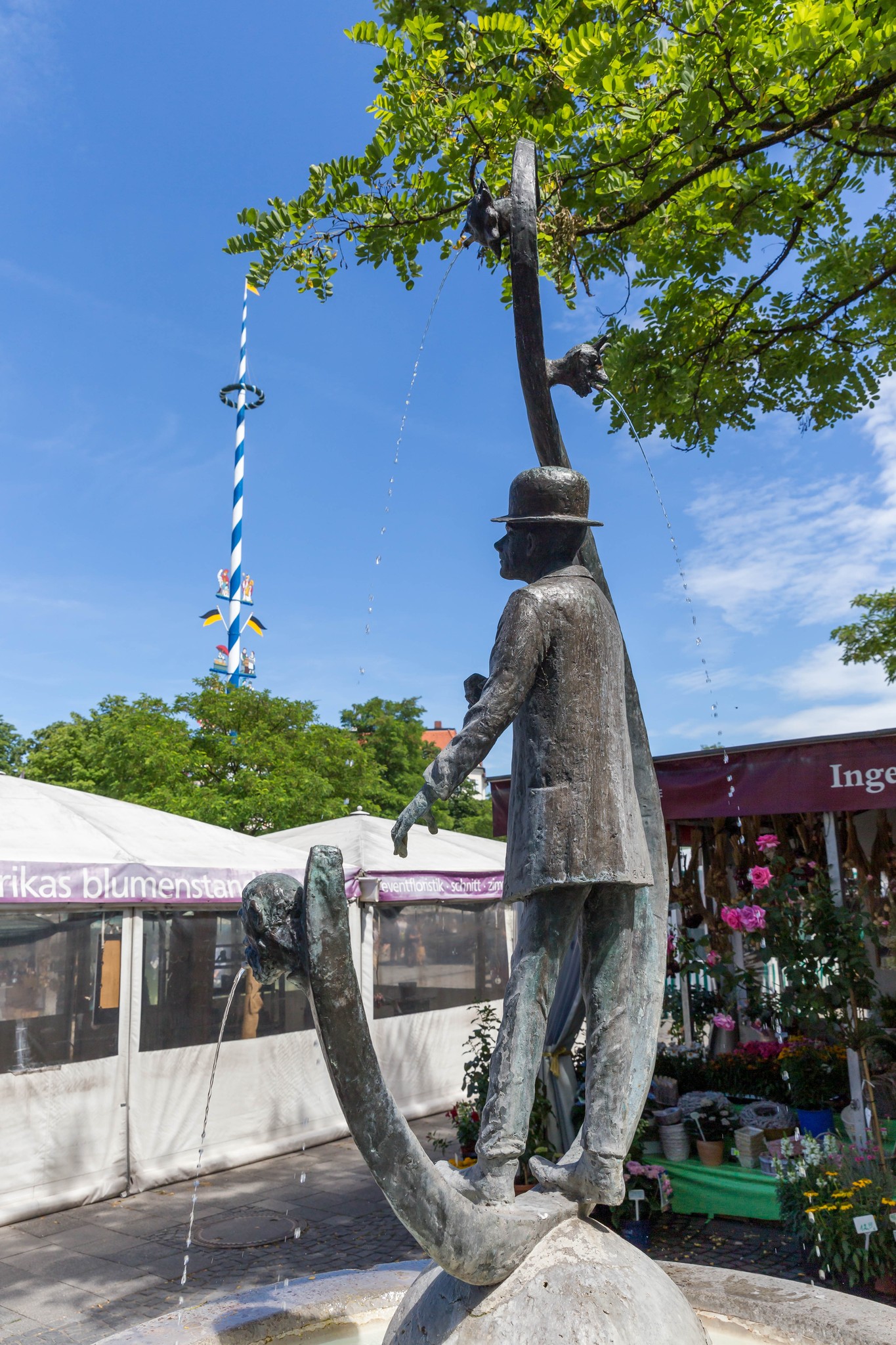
<box><xmin>391</xmin><ymin>464</ymin><xmax>654</xmax><ymax>1208</ymax></box>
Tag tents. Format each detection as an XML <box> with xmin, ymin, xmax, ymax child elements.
<box><xmin>0</xmin><ymin>776</ymin><xmax>367</xmax><ymax>1225</ymax></box>
<box><xmin>257</xmin><ymin>803</ymin><xmax>522</xmax><ymax>1121</ymax></box>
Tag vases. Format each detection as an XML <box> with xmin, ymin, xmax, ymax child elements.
<box><xmin>697</xmin><ymin>1139</ymin><xmax>726</xmax><ymax>1166</ymax></box>
<box><xmin>734</xmin><ymin>1127</ymin><xmax>765</xmax><ymax>1168</ymax></box>
<box><xmin>658</xmin><ymin>1125</ymin><xmax>690</xmax><ymax>1161</ymax></box>
<box><xmin>796</xmin><ymin>1108</ymin><xmax>834</xmax><ymax>1138</ymax></box>
<box><xmin>514</xmin><ymin>1175</ymin><xmax>536</xmax><ymax>1196</ymax></box>
<box><xmin>874</xmin><ymin>1271</ymin><xmax>896</xmax><ymax>1294</ymax></box>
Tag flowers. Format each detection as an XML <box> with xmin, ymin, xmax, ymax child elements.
<box><xmin>424</xmin><ymin>830</ymin><xmax>896</xmax><ymax>1298</ymax></box>
